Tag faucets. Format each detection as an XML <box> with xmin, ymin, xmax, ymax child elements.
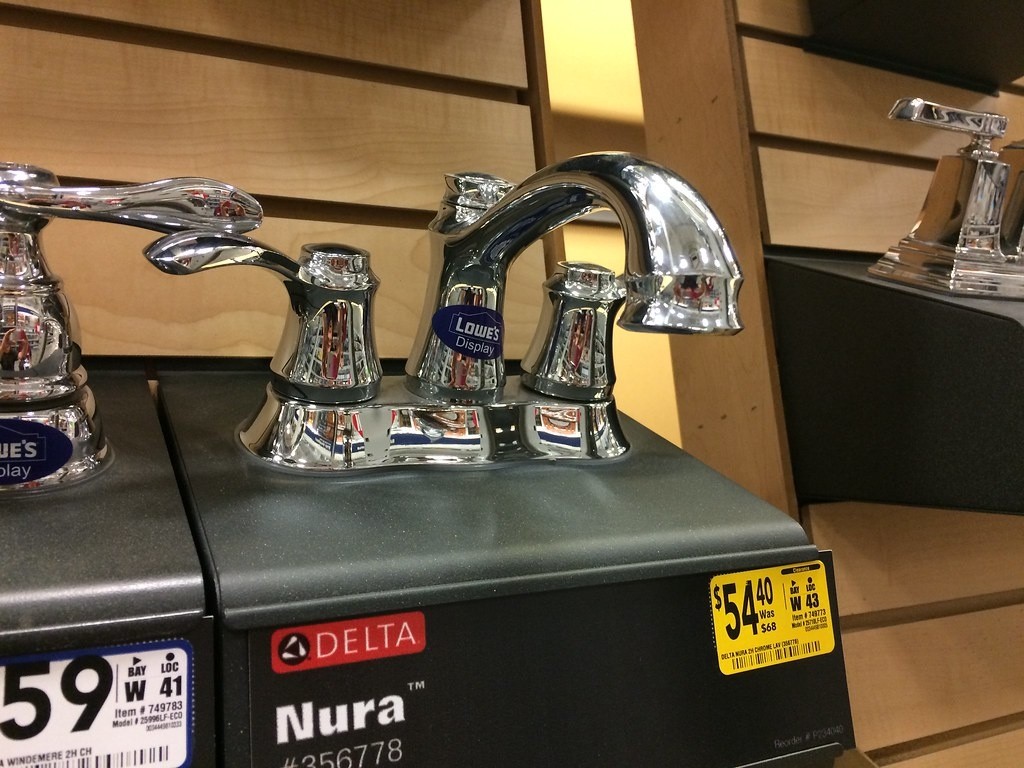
<box><xmin>404</xmin><ymin>150</ymin><xmax>743</xmax><ymax>404</ymax></box>
